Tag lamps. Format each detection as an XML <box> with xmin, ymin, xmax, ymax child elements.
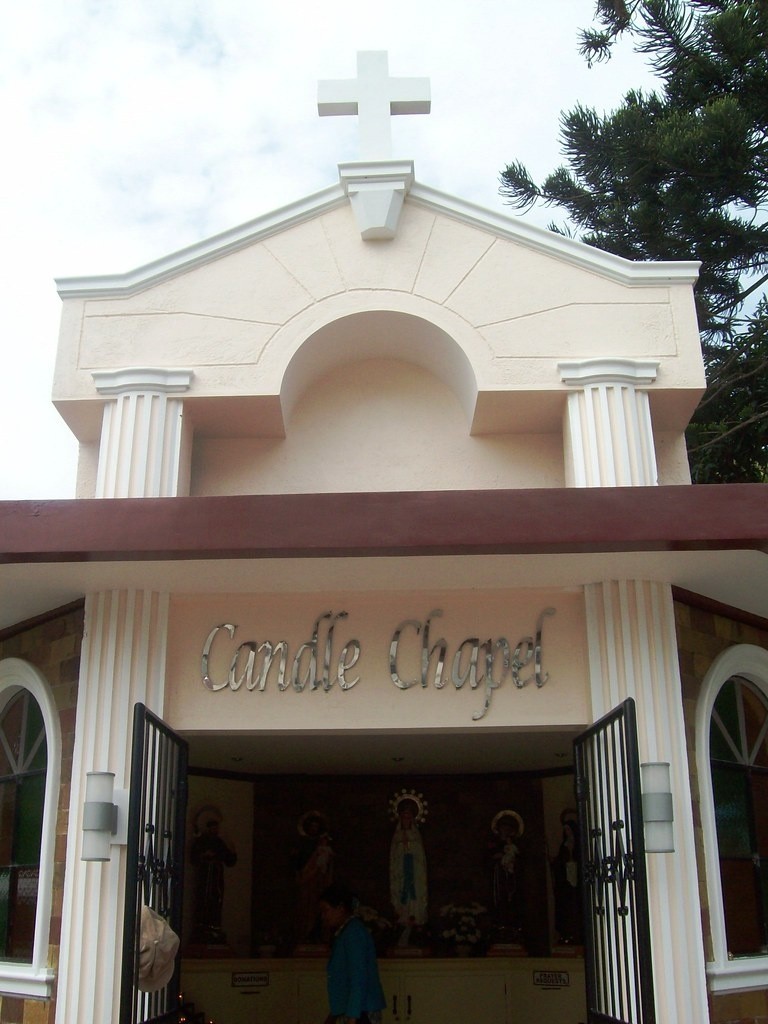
<box><xmin>640</xmin><ymin>762</ymin><xmax>675</xmax><ymax>853</ymax></box>
<box><xmin>81</xmin><ymin>771</ymin><xmax>118</xmax><ymax>861</ymax></box>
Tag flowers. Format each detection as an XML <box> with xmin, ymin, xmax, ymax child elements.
<box><xmin>354</xmin><ymin>907</ymin><xmax>393</xmax><ymax>930</ymax></box>
<box><xmin>441</xmin><ymin>901</ymin><xmax>488</xmax><ymax>943</ymax></box>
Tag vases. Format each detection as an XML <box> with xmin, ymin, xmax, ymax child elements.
<box><xmin>456</xmin><ymin>945</ymin><xmax>471</xmax><ymax>958</ymax></box>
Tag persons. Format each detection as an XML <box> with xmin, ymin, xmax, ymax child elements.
<box><xmin>189</xmin><ymin>809</ymin><xmax>584</xmax><ymax>940</ymax></box>
<box><xmin>319</xmin><ymin>884</ymin><xmax>387</xmax><ymax>1024</ymax></box>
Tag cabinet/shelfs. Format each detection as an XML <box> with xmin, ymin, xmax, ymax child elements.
<box><xmin>296</xmin><ymin>957</ymin><xmax>507</xmax><ymax>1024</ymax></box>
